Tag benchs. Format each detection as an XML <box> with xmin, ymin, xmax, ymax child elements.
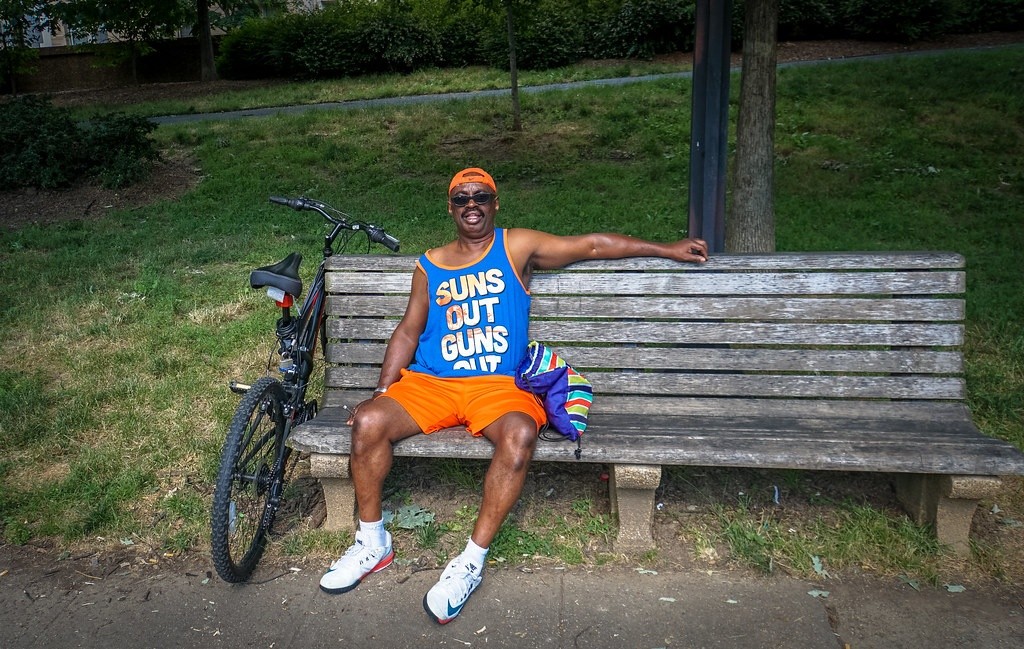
<box><xmin>285</xmin><ymin>252</ymin><xmax>1024</xmax><ymax>558</ymax></box>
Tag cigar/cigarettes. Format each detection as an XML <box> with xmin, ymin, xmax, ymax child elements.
<box><xmin>343</xmin><ymin>406</ymin><xmax>355</xmax><ymax>416</ymax></box>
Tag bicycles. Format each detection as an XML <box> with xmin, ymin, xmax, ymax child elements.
<box><xmin>206</xmin><ymin>190</ymin><xmax>401</xmax><ymax>583</ymax></box>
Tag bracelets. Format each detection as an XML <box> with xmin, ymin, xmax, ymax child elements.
<box><xmin>374</xmin><ymin>388</ymin><xmax>387</xmax><ymax>393</ymax></box>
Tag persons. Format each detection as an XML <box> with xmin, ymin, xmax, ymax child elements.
<box><xmin>319</xmin><ymin>168</ymin><xmax>708</xmax><ymax>625</ymax></box>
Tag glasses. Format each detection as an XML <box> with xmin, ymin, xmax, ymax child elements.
<box><xmin>452</xmin><ymin>192</ymin><xmax>499</xmax><ymax>207</ymax></box>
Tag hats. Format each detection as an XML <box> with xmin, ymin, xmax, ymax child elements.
<box><xmin>448</xmin><ymin>168</ymin><xmax>496</xmax><ymax>195</ymax></box>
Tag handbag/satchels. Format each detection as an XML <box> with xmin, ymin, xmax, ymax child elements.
<box><xmin>514</xmin><ymin>339</ymin><xmax>594</xmax><ymax>460</ymax></box>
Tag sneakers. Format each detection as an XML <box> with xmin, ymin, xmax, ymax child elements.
<box><xmin>318</xmin><ymin>530</ymin><xmax>395</xmax><ymax>594</ymax></box>
<box><xmin>423</xmin><ymin>552</ymin><xmax>483</xmax><ymax>626</ymax></box>
